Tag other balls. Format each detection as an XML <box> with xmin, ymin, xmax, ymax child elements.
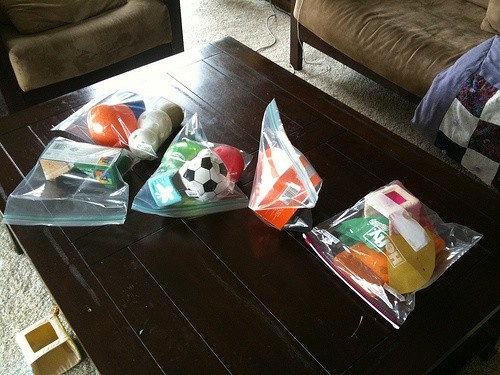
<box><xmin>128</xmin><ymin>128</ymin><xmax>159</xmax><ymax>159</ymax></box>
<box><xmin>138</xmin><ymin>109</ymin><xmax>173</xmax><ymax>143</ymax></box>
<box><xmin>160</xmin><ymin>101</ymin><xmax>184</xmax><ymax>129</ymax></box>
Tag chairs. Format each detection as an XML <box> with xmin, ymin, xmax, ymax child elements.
<box><xmin>1</xmin><ymin>0</ymin><xmax>184</xmax><ymax>115</ymax></box>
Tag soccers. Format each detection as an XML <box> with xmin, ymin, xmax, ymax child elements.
<box><xmin>178</xmin><ymin>147</ymin><xmax>230</xmax><ymax>203</ymax></box>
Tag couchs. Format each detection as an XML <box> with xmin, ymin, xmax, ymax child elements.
<box><xmin>289</xmin><ymin>0</ymin><xmax>500</xmax><ymax>187</ymax></box>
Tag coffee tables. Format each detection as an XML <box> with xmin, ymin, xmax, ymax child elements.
<box><xmin>0</xmin><ymin>37</ymin><xmax>499</xmax><ymax>375</ymax></box>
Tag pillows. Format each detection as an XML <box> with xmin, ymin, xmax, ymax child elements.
<box><xmin>480</xmin><ymin>1</ymin><xmax>500</xmax><ymax>35</ymax></box>
